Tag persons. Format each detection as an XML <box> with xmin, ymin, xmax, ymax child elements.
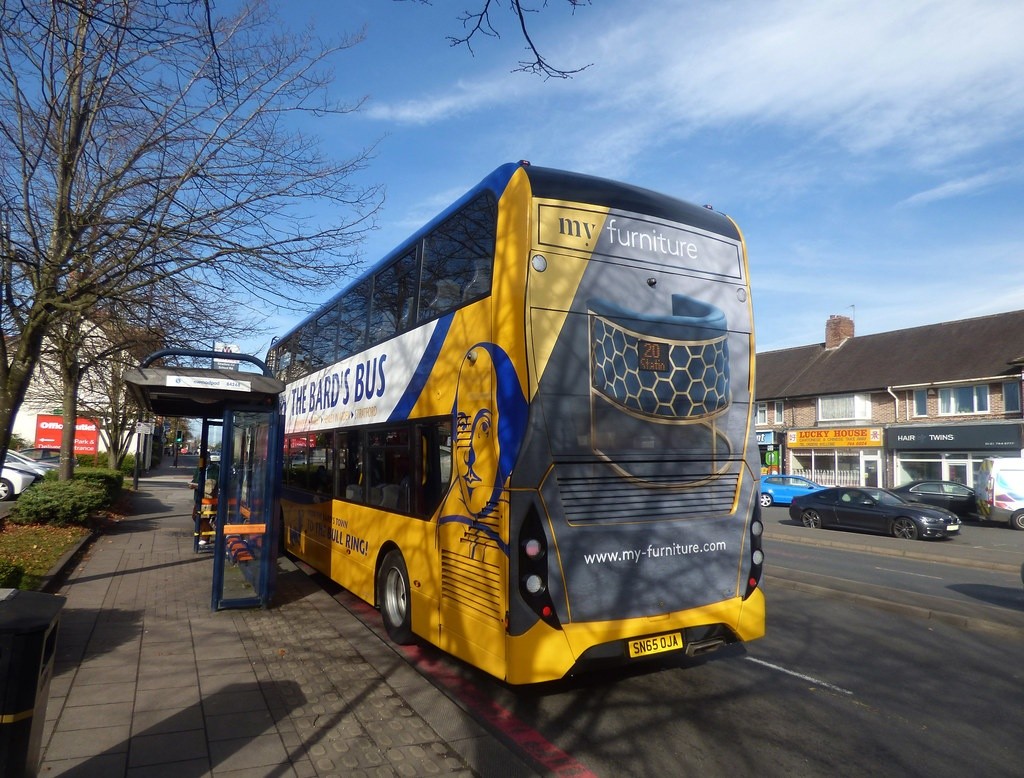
<box><xmin>189</xmin><ymin>452</ymin><xmax>219</xmax><ymax>544</ymax></box>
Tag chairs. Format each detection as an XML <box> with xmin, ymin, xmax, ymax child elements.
<box><xmin>338</xmin><ymin>478</ymin><xmax>415</xmax><ymax>514</ymax></box>
<box><xmin>353</xmin><ymin>254</ymin><xmax>493</xmax><ymax>352</ymax></box>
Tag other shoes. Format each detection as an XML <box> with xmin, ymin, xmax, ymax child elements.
<box><xmin>198</xmin><ymin>540</ymin><xmax>208</xmax><ymax>546</ymax></box>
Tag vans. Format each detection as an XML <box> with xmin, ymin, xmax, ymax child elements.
<box><xmin>976</xmin><ymin>455</ymin><xmax>1024</xmax><ymax>531</ymax></box>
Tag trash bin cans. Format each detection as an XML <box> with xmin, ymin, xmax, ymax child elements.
<box><xmin>0</xmin><ymin>587</ymin><xmax>67</xmax><ymax>778</ymax></box>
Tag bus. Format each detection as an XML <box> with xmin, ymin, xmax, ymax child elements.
<box><xmin>256</xmin><ymin>159</ymin><xmax>767</xmax><ymax>689</ymax></box>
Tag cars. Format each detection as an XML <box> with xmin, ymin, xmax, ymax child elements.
<box><xmin>788</xmin><ymin>486</ymin><xmax>963</xmax><ymax>541</ymax></box>
<box><xmin>0</xmin><ymin>447</ymin><xmax>81</xmax><ymax>502</ymax></box>
<box><xmin>210</xmin><ymin>452</ymin><xmax>222</xmax><ymax>462</ymax></box>
<box><xmin>760</xmin><ymin>473</ymin><xmax>832</xmax><ymax>507</ymax></box>
<box><xmin>887</xmin><ymin>479</ymin><xmax>979</xmax><ymax>520</ymax></box>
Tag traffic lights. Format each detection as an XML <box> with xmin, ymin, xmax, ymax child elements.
<box><xmin>177</xmin><ymin>430</ymin><xmax>182</xmax><ymax>443</ymax></box>
